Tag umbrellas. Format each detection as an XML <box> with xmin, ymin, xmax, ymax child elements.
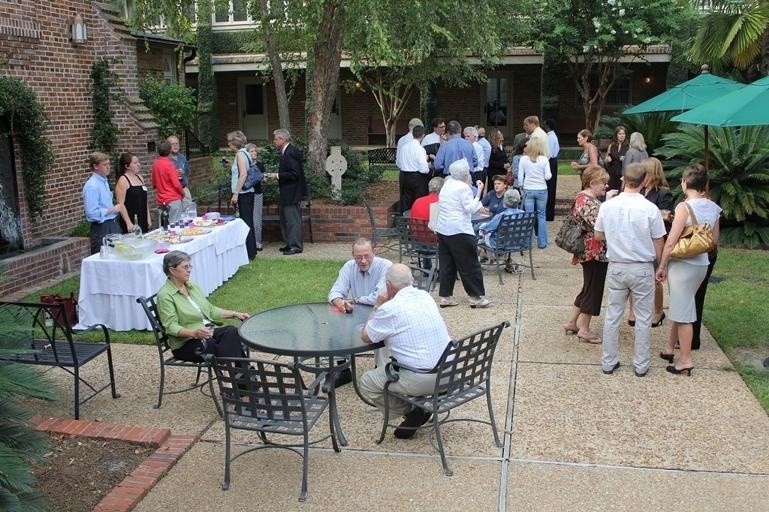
<box><xmin>670</xmin><ymin>75</ymin><xmax>769</xmax><ymax>126</ymax></box>
<box><xmin>622</xmin><ymin>65</ymin><xmax>749</xmax><ymax>199</ymax></box>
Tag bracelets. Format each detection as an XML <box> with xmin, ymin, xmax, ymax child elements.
<box><xmin>373</xmin><ymin>306</ymin><xmax>378</xmax><ymax>312</ymax></box>
<box><xmin>233</xmin><ymin>311</ymin><xmax>235</xmax><ymax>320</ymax></box>
<box><xmin>428</xmin><ymin>154</ymin><xmax>430</xmax><ymax>161</ymax></box>
<box><xmin>113</xmin><ymin>206</ymin><xmax>120</xmax><ymax>212</ymax></box>
<box><xmin>353</xmin><ymin>296</ymin><xmax>358</xmax><ymax>304</ymax></box>
<box><xmin>235</xmin><ymin>192</ymin><xmax>239</xmax><ymax>195</ymax></box>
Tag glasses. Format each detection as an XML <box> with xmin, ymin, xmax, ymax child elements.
<box><xmin>435</xmin><ymin>126</ymin><xmax>446</xmax><ymax>128</ymax></box>
<box><xmin>173</xmin><ymin>265</ymin><xmax>193</xmax><ymax>270</ymax></box>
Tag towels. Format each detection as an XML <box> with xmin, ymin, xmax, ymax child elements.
<box><xmin>201</xmin><ymin>215</ymin><xmax>209</xmax><ymax>220</ymax></box>
<box><xmin>169</xmin><ymin>230</ymin><xmax>177</xmax><ymax>235</ymax></box>
<box><xmin>153</xmin><ymin>247</ymin><xmax>170</xmax><ymax>254</ymax></box>
<box><xmin>216</xmin><ymin>218</ymin><xmax>228</xmax><ymax>224</ymax></box>
<box><xmin>187</xmin><ymin>222</ymin><xmax>196</xmax><ymax>228</ymax></box>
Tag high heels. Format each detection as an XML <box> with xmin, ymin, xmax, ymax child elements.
<box><xmin>651</xmin><ymin>312</ymin><xmax>665</xmax><ymax>327</ymax></box>
<box><xmin>628</xmin><ymin>320</ymin><xmax>635</xmax><ymax>325</ymax></box>
<box><xmin>564</xmin><ymin>326</ymin><xmax>579</xmax><ymax>336</ymax></box>
<box><xmin>667</xmin><ymin>366</ymin><xmax>694</xmax><ymax>376</ymax></box>
<box><xmin>578</xmin><ymin>332</ymin><xmax>602</xmax><ymax>343</ymax></box>
<box><xmin>660</xmin><ymin>352</ymin><xmax>674</xmax><ymax>363</ymax></box>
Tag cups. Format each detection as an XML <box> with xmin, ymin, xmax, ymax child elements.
<box><xmin>344</xmin><ymin>299</ymin><xmax>354</xmax><ymax>315</ymax></box>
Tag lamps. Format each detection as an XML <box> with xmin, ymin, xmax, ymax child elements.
<box><xmin>68</xmin><ymin>11</ymin><xmax>87</xmax><ymax>44</ymax></box>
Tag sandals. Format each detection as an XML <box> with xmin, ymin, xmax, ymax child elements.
<box><xmin>440</xmin><ymin>297</ymin><xmax>459</xmax><ymax>308</ymax></box>
<box><xmin>467</xmin><ymin>296</ymin><xmax>492</xmax><ymax>308</ymax></box>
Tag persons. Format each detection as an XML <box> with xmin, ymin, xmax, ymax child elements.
<box><xmin>152</xmin><ymin>143</ymin><xmax>185</xmax><ymax>225</ymax></box>
<box><xmin>593</xmin><ymin>162</ymin><xmax>666</xmax><ymax>377</ymax></box>
<box><xmin>514</xmin><ymin>118</ymin><xmax>530</xmax><ymax>155</ymax></box>
<box><xmin>487</xmin><ymin>129</ymin><xmax>511</xmax><ymax>192</ymax></box>
<box><xmin>481</xmin><ymin>175</ymin><xmax>508</xmax><ymax>214</ymax></box>
<box><xmin>511</xmin><ymin>139</ymin><xmax>531</xmax><ymax>189</ymax></box>
<box><xmin>477</xmin><ymin>127</ymin><xmax>492</xmax><ymax>199</ymax></box>
<box><xmin>394</xmin><ymin>118</ymin><xmax>423</xmax><ymax>210</ymax></box>
<box><xmin>541</xmin><ymin>118</ymin><xmax>560</xmax><ymax>222</ymax></box>
<box><xmin>400</xmin><ymin>125</ymin><xmax>431</xmax><ymax>241</ymax></box>
<box><xmin>571</xmin><ymin>130</ymin><xmax>599</xmax><ymax>190</ymax></box>
<box><xmin>674</xmin><ymin>249</ymin><xmax>717</xmax><ymax>350</ymax></box>
<box><xmin>360</xmin><ymin>263</ymin><xmax>456</xmax><ymax>439</ymax></box>
<box><xmin>473</xmin><ymin>189</ymin><xmax>529</xmax><ymax>263</ymax></box>
<box><xmin>115</xmin><ymin>152</ymin><xmax>152</xmax><ymax>234</ymax></box>
<box><xmin>221</xmin><ymin>131</ymin><xmax>257</xmax><ymax>259</ymax></box>
<box><xmin>157</xmin><ymin>251</ymin><xmax>262</xmax><ymax>398</ymax></box>
<box><xmin>321</xmin><ymin>237</ymin><xmax>393</xmax><ymax>393</ymax></box>
<box><xmin>430</xmin><ymin>121</ymin><xmax>478</xmax><ymax>186</ymax></box>
<box><xmin>435</xmin><ymin>158</ymin><xmax>493</xmax><ymax>309</ymax></box>
<box><xmin>629</xmin><ymin>157</ymin><xmax>674</xmax><ymax>327</ymax></box>
<box><xmin>619</xmin><ymin>130</ymin><xmax>649</xmax><ymax>192</ymax></box>
<box><xmin>410</xmin><ymin>176</ymin><xmax>446</xmax><ymax>281</ymax></box>
<box><xmin>420</xmin><ymin>117</ymin><xmax>448</xmax><ymax>177</ymax></box>
<box><xmin>245</xmin><ymin>142</ymin><xmax>267</xmax><ymax>251</ymax></box>
<box><xmin>523</xmin><ymin>116</ymin><xmax>552</xmax><ymax>238</ymax></box>
<box><xmin>564</xmin><ymin>162</ymin><xmax>619</xmax><ymax>344</ymax></box>
<box><xmin>167</xmin><ymin>135</ymin><xmax>192</xmax><ymax>216</ymax></box>
<box><xmin>655</xmin><ymin>164</ymin><xmax>723</xmax><ymax>378</ymax></box>
<box><xmin>604</xmin><ymin>125</ymin><xmax>629</xmax><ymax>189</ymax></box>
<box><xmin>265</xmin><ymin>129</ymin><xmax>307</xmax><ymax>255</ymax></box>
<box><xmin>462</xmin><ymin>125</ymin><xmax>485</xmax><ymax>199</ymax></box>
<box><xmin>82</xmin><ymin>152</ymin><xmax>121</xmax><ymax>255</ymax></box>
<box><xmin>518</xmin><ymin>137</ymin><xmax>552</xmax><ymax>249</ymax></box>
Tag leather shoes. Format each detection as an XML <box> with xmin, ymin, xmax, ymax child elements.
<box><xmin>635</xmin><ymin>369</ymin><xmax>649</xmax><ymax>377</ymax></box>
<box><xmin>279</xmin><ymin>246</ymin><xmax>289</xmax><ymax>251</ymax></box>
<box><xmin>282</xmin><ymin>246</ymin><xmax>303</xmax><ymax>255</ymax></box>
<box><xmin>603</xmin><ymin>361</ymin><xmax>620</xmax><ymax>374</ymax></box>
<box><xmin>394</xmin><ymin>408</ymin><xmax>431</xmax><ymax>438</ymax></box>
<box><xmin>323</xmin><ymin>369</ymin><xmax>352</xmax><ymax>393</ymax></box>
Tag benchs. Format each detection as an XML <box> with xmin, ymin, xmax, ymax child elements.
<box><xmin>207</xmin><ymin>185</ymin><xmax>313</xmax><ymax>243</ymax></box>
<box><xmin>368</xmin><ymin>148</ymin><xmax>399</xmax><ymax>172</ymax></box>
<box><xmin>0</xmin><ymin>301</ymin><xmax>120</xmax><ymax>420</ymax></box>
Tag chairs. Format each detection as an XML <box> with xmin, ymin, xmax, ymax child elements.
<box><xmin>205</xmin><ymin>353</ymin><xmax>341</xmax><ymax>502</ymax></box>
<box><xmin>136</xmin><ymin>293</ymin><xmax>249</xmax><ymax>415</ymax></box>
<box><xmin>363</xmin><ymin>198</ymin><xmax>402</xmax><ymax>257</ymax></box>
<box><xmin>477</xmin><ymin>211</ymin><xmax>540</xmax><ymax>285</ymax></box>
<box><xmin>395</xmin><ymin>217</ymin><xmax>442</xmax><ymax>290</ymax></box>
<box><xmin>375</xmin><ymin>320</ymin><xmax>511</xmax><ymax>475</ymax></box>
<box><xmin>405</xmin><ymin>263</ymin><xmax>435</xmax><ymax>293</ymax></box>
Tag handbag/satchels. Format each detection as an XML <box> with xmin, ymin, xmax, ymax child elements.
<box><xmin>238</xmin><ymin>165</ymin><xmax>264</xmax><ymax>190</ymax></box>
<box><xmin>670</xmin><ymin>223</ymin><xmax>715</xmax><ymax>259</ymax></box>
<box><xmin>555</xmin><ymin>212</ymin><xmax>594</xmax><ymax>254</ymax></box>
<box><xmin>40</xmin><ymin>292</ymin><xmax>78</xmax><ymax>326</ymax></box>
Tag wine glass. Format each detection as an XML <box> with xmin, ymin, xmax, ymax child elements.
<box><xmin>212</xmin><ymin>215</ymin><xmax>217</xmax><ymax>224</ymax></box>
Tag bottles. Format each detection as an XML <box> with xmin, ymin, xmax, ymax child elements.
<box><xmin>108</xmin><ymin>244</ymin><xmax>138</xmax><ymax>260</ymax></box>
<box><xmin>161</xmin><ymin>202</ymin><xmax>169</xmax><ymax>229</ymax></box>
<box><xmin>107</xmin><ymin>237</ymin><xmax>136</xmax><ymax>252</ymax></box>
<box><xmin>132</xmin><ymin>214</ymin><xmax>145</xmax><ymax>236</ymax></box>
